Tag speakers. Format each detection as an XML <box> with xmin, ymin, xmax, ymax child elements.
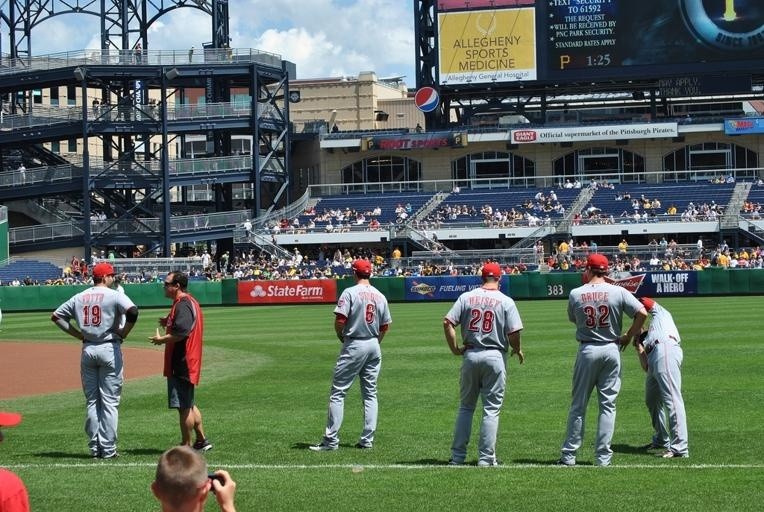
<box><xmin>73</xmin><ymin>68</ymin><xmax>85</xmax><ymax>81</ymax></box>
<box><xmin>165</xmin><ymin>68</ymin><xmax>179</xmax><ymax>80</ymax></box>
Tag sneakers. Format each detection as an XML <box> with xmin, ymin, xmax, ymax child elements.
<box><xmin>552</xmin><ymin>460</ymin><xmax>567</xmax><ymax>464</ymax></box>
<box><xmin>639</xmin><ymin>444</ymin><xmax>667</xmax><ymax>453</ymax></box>
<box><xmin>479</xmin><ymin>460</ymin><xmax>502</xmax><ymax>466</ymax></box>
<box><xmin>193</xmin><ymin>440</ymin><xmax>212</xmax><ymax>452</ymax></box>
<box><xmin>355</xmin><ymin>443</ymin><xmax>372</xmax><ymax>448</ymax></box>
<box><xmin>309</xmin><ymin>443</ymin><xmax>338</xmax><ymax>451</ymax></box>
<box><xmin>662</xmin><ymin>450</ymin><xmax>689</xmax><ymax>458</ymax></box>
<box><xmin>449</xmin><ymin>460</ymin><xmax>457</xmax><ymax>465</ymax></box>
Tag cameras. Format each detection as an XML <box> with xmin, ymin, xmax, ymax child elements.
<box><xmin>208</xmin><ymin>475</ymin><xmax>225</xmax><ymax>491</ymax></box>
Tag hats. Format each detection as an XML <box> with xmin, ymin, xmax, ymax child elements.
<box><xmin>483</xmin><ymin>263</ymin><xmax>501</xmax><ymax>276</ymax></box>
<box><xmin>583</xmin><ymin>255</ymin><xmax>608</xmax><ymax>270</ymax></box>
<box><xmin>93</xmin><ymin>263</ymin><xmax>120</xmax><ymax>277</ymax></box>
<box><xmin>0</xmin><ymin>414</ymin><xmax>20</xmax><ymax>425</ymax></box>
<box><xmin>352</xmin><ymin>261</ymin><xmax>371</xmax><ymax>273</ymax></box>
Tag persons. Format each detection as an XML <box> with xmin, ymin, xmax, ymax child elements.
<box><xmin>442</xmin><ymin>262</ymin><xmax>525</xmax><ymax>467</ymax></box>
<box><xmin>555</xmin><ymin>253</ymin><xmax>648</xmax><ymax>467</ymax></box>
<box><xmin>134</xmin><ymin>42</ymin><xmax>143</xmax><ymax>65</ymax></box>
<box><xmin>147</xmin><ymin>271</ymin><xmax>214</xmax><ymax>451</ymax></box>
<box><xmin>188</xmin><ymin>46</ymin><xmax>194</xmax><ymax>63</ymax></box>
<box><xmin>213</xmin><ymin>148</ymin><xmax>244</xmax><ymax>171</ymax></box>
<box><xmin>1</xmin><ymin>411</ymin><xmax>31</xmax><ymax>512</ymax></box>
<box><xmin>332</xmin><ymin>123</ymin><xmax>338</xmax><ymax>132</ymax></box>
<box><xmin>113</xmin><ymin>277</ymin><xmax>130</xmax><ymax>331</ymax></box>
<box><xmin>1</xmin><ymin>249</ymin><xmax>241</xmax><ymax>288</ymax></box>
<box><xmin>241</xmin><ymin>171</ymin><xmax>764</xmax><ymax>282</ymax></box>
<box><xmin>89</xmin><ymin>208</ymin><xmax>212</xmax><ymax>233</ymax></box>
<box><xmin>308</xmin><ymin>259</ymin><xmax>393</xmax><ymax>452</ymax></box>
<box><xmin>49</xmin><ymin>262</ymin><xmax>138</xmax><ymax>459</ymax></box>
<box><xmin>632</xmin><ymin>296</ymin><xmax>691</xmax><ymax>459</ymax></box>
<box><xmin>149</xmin><ymin>445</ymin><xmax>236</xmax><ymax>511</ymax></box>
<box><xmin>223</xmin><ymin>42</ymin><xmax>234</xmax><ymax>64</ymax></box>
<box><xmin>6</xmin><ymin>164</ymin><xmax>13</xmax><ymax>186</ymax></box>
<box><xmin>92</xmin><ymin>94</ymin><xmax>162</xmax><ymax>121</ymax></box>
<box><xmin>415</xmin><ymin>122</ymin><xmax>423</xmax><ymax>133</ymax></box>
<box><xmin>16</xmin><ymin>163</ymin><xmax>27</xmax><ymax>186</ymax></box>
